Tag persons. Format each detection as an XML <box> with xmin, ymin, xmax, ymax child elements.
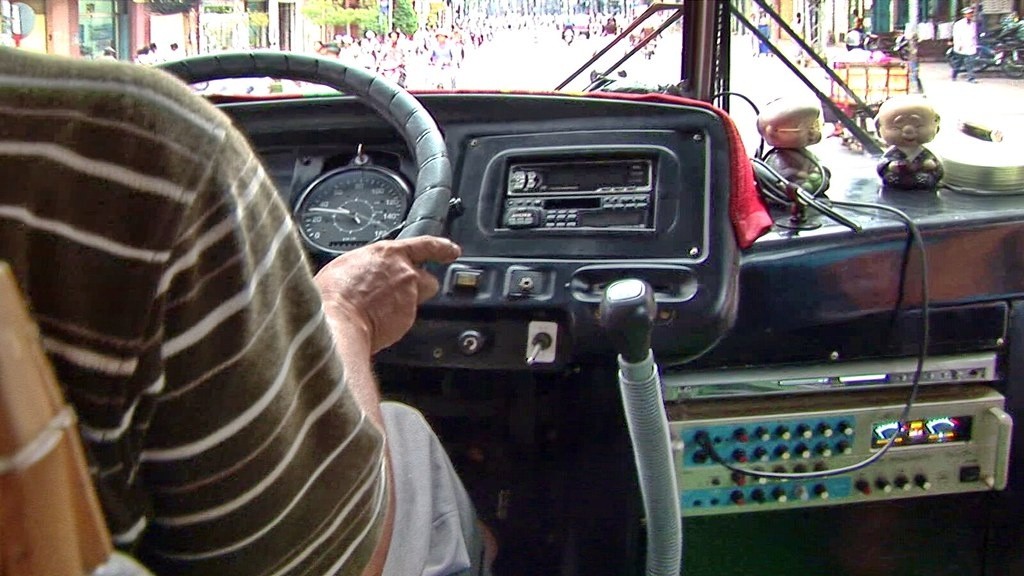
<box><xmin>756</xmin><ymin>96</ymin><xmax>831</xmax><ymax>196</ymax></box>
<box><xmin>0</xmin><ymin>40</ymin><xmax>498</xmax><ymax>576</ymax></box>
<box><xmin>845</xmin><ymin>8</ymin><xmax>868</xmax><ymax>51</ymax></box>
<box><xmin>875</xmin><ymin>95</ymin><xmax>945</xmax><ymax>190</ymax></box>
<box><xmin>326</xmin><ymin>10</ymin><xmax>663</xmax><ymax>93</ymax></box>
<box><xmin>792</xmin><ymin>11</ymin><xmax>804</xmax><ymax>37</ymax></box>
<box><xmin>950</xmin><ymin>6</ymin><xmax>980</xmax><ymax>84</ymax></box>
<box><xmin>101</xmin><ymin>39</ymin><xmax>187</xmax><ymax>69</ymax></box>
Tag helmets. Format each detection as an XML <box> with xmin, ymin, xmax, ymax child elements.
<box><xmin>845</xmin><ymin>30</ymin><xmax>864</xmax><ymax>47</ymax></box>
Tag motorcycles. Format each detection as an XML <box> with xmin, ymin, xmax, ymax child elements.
<box><xmin>864</xmin><ymin>30</ymin><xmax>915</xmax><ymax>62</ymax></box>
<box><xmin>836</xmin><ymin>99</ymin><xmax>884</xmax><ymax>155</ymax></box>
<box><xmin>945</xmin><ymin>26</ymin><xmax>1024</xmax><ymax>80</ymax></box>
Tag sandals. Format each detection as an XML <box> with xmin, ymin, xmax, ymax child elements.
<box><xmin>447</xmin><ymin>429</ymin><xmax>521</xmax><ymax>576</ymax></box>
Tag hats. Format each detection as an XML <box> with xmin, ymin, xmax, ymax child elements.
<box><xmin>960</xmin><ymin>7</ymin><xmax>974</xmax><ymax>14</ymax></box>
